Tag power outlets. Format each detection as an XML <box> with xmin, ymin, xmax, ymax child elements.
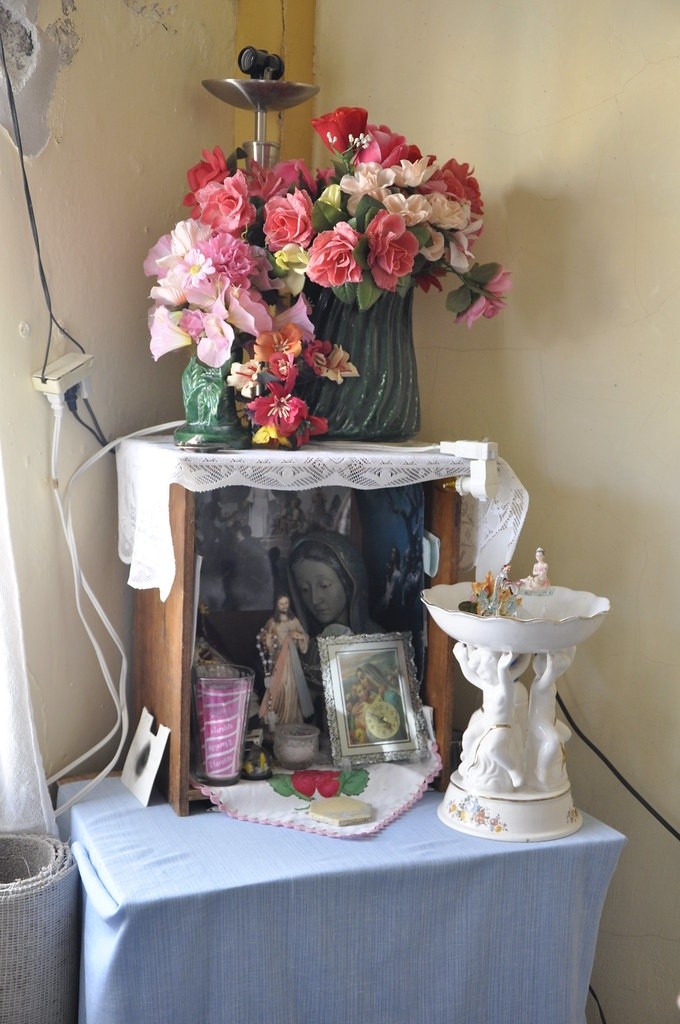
<box><xmin>34</xmin><ymin>353</ymin><xmax>96</xmax><ymax>395</ymax></box>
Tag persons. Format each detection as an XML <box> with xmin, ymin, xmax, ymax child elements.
<box><xmin>529</xmin><ymin>547</ymin><xmax>551</xmax><ymax>587</ymax></box>
<box><xmin>258</xmin><ymin>592</ymin><xmax>314</xmax><ymax>730</ymax></box>
<box><xmin>383</xmin><ymin>546</ymin><xmax>402</xmax><ymax>620</ymax></box>
<box><xmin>285</xmin><ymin>531</ymin><xmax>388</xmax><ymax>703</ymax></box>
<box><xmin>453</xmin><ymin>644</ymin><xmax>524</xmax><ymax>787</ymax></box>
<box><xmin>528</xmin><ymin>645</ymin><xmax>576</xmax><ymax>789</ymax></box>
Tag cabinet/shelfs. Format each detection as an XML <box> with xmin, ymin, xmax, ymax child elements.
<box><xmin>52</xmin><ymin>766</ymin><xmax>628</xmax><ymax>1024</ymax></box>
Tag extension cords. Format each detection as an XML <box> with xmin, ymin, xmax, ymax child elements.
<box><xmin>32</xmin><ymin>352</ymin><xmax>94</xmax><ymax>393</ymax></box>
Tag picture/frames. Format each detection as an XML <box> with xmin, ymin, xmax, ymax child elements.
<box><xmin>315</xmin><ymin>632</ymin><xmax>435</xmax><ymax>770</ymax></box>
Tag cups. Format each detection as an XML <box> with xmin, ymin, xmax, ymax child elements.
<box><xmin>191</xmin><ymin>663</ymin><xmax>256</xmax><ymax>787</ymax></box>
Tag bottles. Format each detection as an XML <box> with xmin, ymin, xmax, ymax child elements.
<box><xmin>272</xmin><ymin>724</ymin><xmax>321</xmax><ymax>770</ymax></box>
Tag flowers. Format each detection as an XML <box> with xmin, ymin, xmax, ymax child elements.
<box><xmin>173</xmin><ymin>247</ymin><xmax>422</xmax><ymax>447</ymax></box>
<box><xmin>144</xmin><ymin>102</ymin><xmax>511</xmax><ymax>453</ymax></box>
